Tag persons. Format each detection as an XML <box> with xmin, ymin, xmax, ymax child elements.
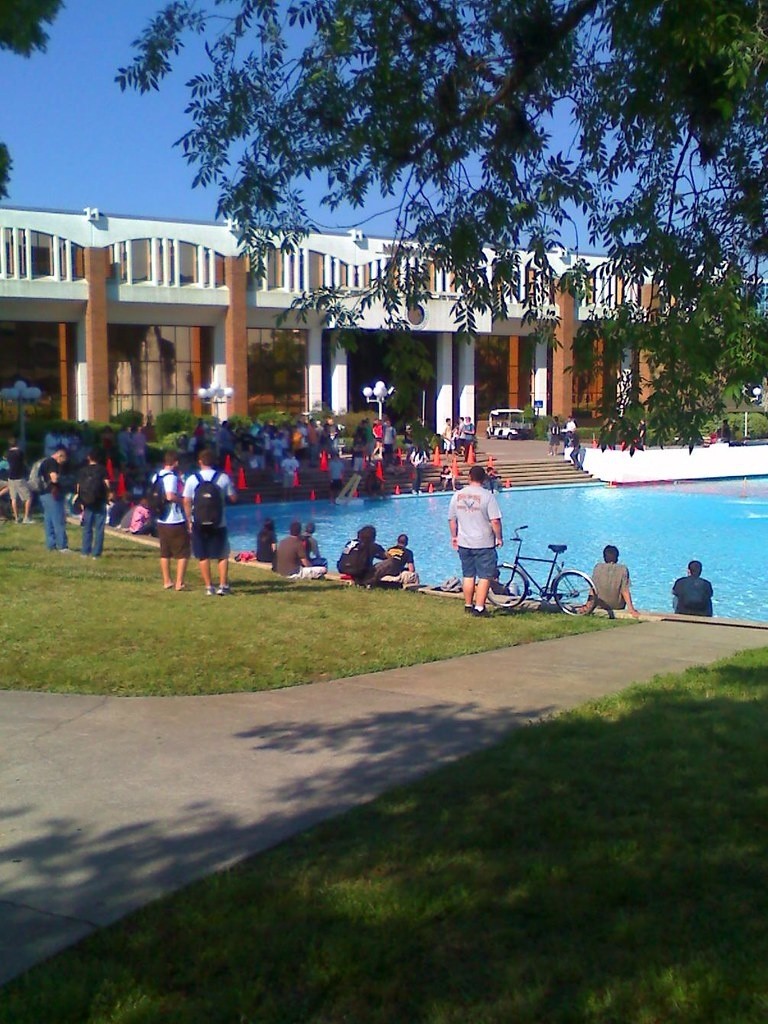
<box><xmin>150</xmin><ymin>447</ymin><xmax>239</xmax><ymax>595</ymax></box>
<box><xmin>336</xmin><ymin>526</ymin><xmax>418</xmax><ymax>584</ymax></box>
<box><xmin>443</xmin><ymin>417</ymin><xmax>475</xmax><ymax>457</ymax></box>
<box><xmin>475</xmin><ymin>570</ymin><xmax>510</xmax><ymax>595</ymax></box>
<box><xmin>0</xmin><ymin>434</ymin><xmax>34</xmax><ymax>523</ymax></box>
<box><xmin>38</xmin><ymin>447</ymin><xmax>75</xmax><ymax>553</ymax></box>
<box><xmin>722</xmin><ymin>420</ymin><xmax>730</xmax><ymax>440</ymax></box>
<box><xmin>440</xmin><ymin>466</ymin><xmax>456</xmax><ymax>492</ymax></box>
<box><xmin>671</xmin><ymin>561</ymin><xmax>714</xmax><ymax>617</ymax></box>
<box><xmin>486</xmin><ymin>467</ymin><xmax>502</xmax><ymax>494</ymax></box>
<box><xmin>638</xmin><ymin>417</ymin><xmax>646</xmax><ymax>445</ymax></box>
<box><xmin>181</xmin><ymin>416</ymin><xmax>345</xmax><ymax>493</ymax></box>
<box><xmin>103</xmin><ymin>423</ymin><xmax>159</xmax><ymax>538</ymax></box>
<box><xmin>76</xmin><ymin>450</ymin><xmax>110</xmax><ymax>558</ymax></box>
<box><xmin>353</xmin><ymin>416</ymin><xmax>430</xmax><ymax>493</ymax></box>
<box><xmin>579</xmin><ymin>545</ymin><xmax>639</xmax><ymax>617</ymax></box>
<box><xmin>567</xmin><ymin>431</ymin><xmax>581</xmax><ymax>470</ymax></box>
<box><xmin>257</xmin><ymin>517</ymin><xmax>328</xmax><ymax>580</ymax></box>
<box><xmin>548</xmin><ymin>415</ymin><xmax>578</xmax><ymax>456</ymax></box>
<box><xmin>448</xmin><ymin>466</ymin><xmax>503</xmax><ymax>618</ymax></box>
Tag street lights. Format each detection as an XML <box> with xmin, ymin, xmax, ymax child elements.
<box><xmin>1</xmin><ymin>380</ymin><xmax>41</xmax><ymax>448</ymax></box>
<box><xmin>198</xmin><ymin>383</ymin><xmax>234</xmax><ymax>456</ymax></box>
<box><xmin>363</xmin><ymin>381</ymin><xmax>396</xmax><ymax>420</ymax></box>
<box><xmin>741</xmin><ymin>384</ymin><xmax>761</xmax><ymax>436</ymax></box>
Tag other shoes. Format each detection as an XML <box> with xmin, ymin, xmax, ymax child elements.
<box><xmin>206</xmin><ymin>587</ymin><xmax>213</xmax><ymax>595</ymax></box>
<box><xmin>217</xmin><ymin>587</ymin><xmax>231</xmax><ymax>595</ymax></box>
<box><xmin>473</xmin><ymin>608</ymin><xmax>495</xmax><ymax>618</ymax></box>
<box><xmin>22</xmin><ymin>519</ymin><xmax>36</xmax><ymax>524</ymax></box>
<box><xmin>464</xmin><ymin>606</ymin><xmax>474</xmax><ymax>614</ymax></box>
<box><xmin>59</xmin><ymin>548</ymin><xmax>71</xmax><ymax>553</ymax></box>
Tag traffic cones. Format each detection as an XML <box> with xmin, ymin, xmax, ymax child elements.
<box><xmin>428</xmin><ymin>483</ymin><xmax>434</xmax><ymax>492</ymax></box>
<box><xmin>292</xmin><ymin>470</ymin><xmax>301</xmax><ymax>486</ymax></box>
<box><xmin>116</xmin><ymin>473</ymin><xmax>128</xmax><ymax>497</ymax></box>
<box><xmin>236</xmin><ymin>467</ymin><xmax>248</xmax><ymax>489</ymax></box>
<box><xmin>255</xmin><ymin>494</ymin><xmax>261</xmax><ymax>504</ymax></box>
<box><xmin>506</xmin><ymin>479</ymin><xmax>510</xmax><ymax>488</ymax></box>
<box><xmin>487</xmin><ymin>456</ymin><xmax>495</xmax><ymax>475</ymax></box>
<box><xmin>309</xmin><ymin>490</ymin><xmax>316</xmax><ymax>500</ymax></box>
<box><xmin>354</xmin><ymin>489</ymin><xmax>358</xmax><ymax>497</ymax></box>
<box><xmin>106</xmin><ymin>458</ymin><xmax>115</xmax><ymax>483</ymax></box>
<box><xmin>376</xmin><ymin>461</ymin><xmax>383</xmax><ymax>479</ymax></box>
<box><xmin>223</xmin><ymin>455</ymin><xmax>234</xmax><ymax>476</ymax></box>
<box><xmin>452</xmin><ymin>457</ymin><xmax>459</xmax><ymax>476</ymax></box>
<box><xmin>395</xmin><ymin>485</ymin><xmax>400</xmax><ymax>495</ymax></box>
<box><xmin>319</xmin><ymin>451</ymin><xmax>329</xmax><ymax>471</ymax></box>
<box><xmin>432</xmin><ymin>446</ymin><xmax>440</xmax><ymax>466</ymax></box>
<box><xmin>592</xmin><ymin>440</ymin><xmax>597</xmax><ymax>448</ymax></box>
<box><xmin>467</xmin><ymin>445</ymin><xmax>475</xmax><ymax>463</ymax></box>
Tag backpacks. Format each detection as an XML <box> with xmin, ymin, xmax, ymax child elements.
<box><xmin>194</xmin><ymin>471</ymin><xmax>222</xmax><ymax>528</ymax></box>
<box><xmin>149</xmin><ymin>472</ymin><xmax>173</xmax><ymax>519</ymax></box>
<box><xmin>341</xmin><ymin>538</ymin><xmax>370</xmax><ymax>576</ymax></box>
<box><xmin>79</xmin><ymin>467</ymin><xmax>105</xmax><ymax>505</ymax></box>
<box><xmin>28</xmin><ymin>456</ymin><xmax>56</xmax><ymax>493</ymax></box>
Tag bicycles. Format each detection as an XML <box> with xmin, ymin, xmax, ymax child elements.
<box><xmin>487</xmin><ymin>525</ymin><xmax>598</xmax><ymax>616</ymax></box>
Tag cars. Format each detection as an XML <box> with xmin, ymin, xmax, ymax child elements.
<box><xmin>720</xmin><ymin>439</ymin><xmax>768</xmax><ymax>447</ymax></box>
